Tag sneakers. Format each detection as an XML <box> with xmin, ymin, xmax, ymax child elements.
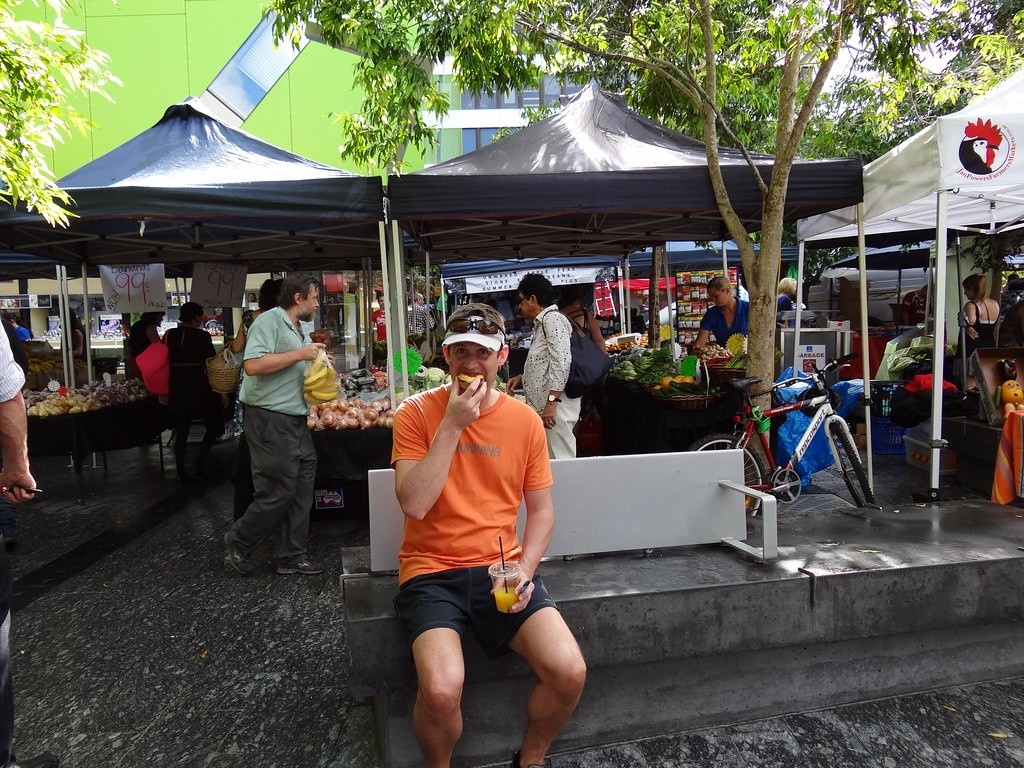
<box><xmin>224</xmin><ymin>533</ymin><xmax>254</xmax><ymax>574</ymax></box>
<box><xmin>276</xmin><ymin>559</ymin><xmax>326</xmax><ymax>575</ymax></box>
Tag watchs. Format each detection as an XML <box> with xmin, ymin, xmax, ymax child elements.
<box><xmin>547</xmin><ymin>395</ymin><xmax>562</xmax><ymax>402</ymax></box>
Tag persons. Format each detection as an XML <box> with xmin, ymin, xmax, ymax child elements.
<box><xmin>0</xmin><ymin>316</ymin><xmax>58</xmax><ymax>768</ymax></box>
<box><xmin>506</xmin><ymin>274</ymin><xmax>581</xmax><ymax>459</ymax></box>
<box><xmin>2</xmin><ymin>312</ymin><xmax>33</xmax><ymax>374</ymax></box>
<box><xmin>955</xmin><ymin>272</ymin><xmax>1024</xmax><ymax>390</ymax></box>
<box><xmin>57</xmin><ymin>307</ymin><xmax>87</xmax><ymax>362</ymax></box>
<box><xmin>560</xmin><ymin>286</ymin><xmax>607</xmax><ymax>420</ymax></box>
<box><xmin>777</xmin><ymin>278</ymin><xmax>805</xmax><ymax>328</ymax></box>
<box><xmin>372</xmin><ymin>293</ymin><xmax>435</xmax><ymax>341</ymax></box>
<box><xmin>388</xmin><ymin>303</ymin><xmax>587</xmax><ymax>768</ymax></box>
<box><xmin>225</xmin><ymin>274</ymin><xmax>324</xmax><ymax>574</ymax></box>
<box><xmin>128</xmin><ymin>312</ymin><xmax>165</xmax><ymax>445</ymax></box>
<box><xmin>901</xmin><ymin>285</ymin><xmax>932</xmax><ymax>324</ymax></box>
<box><xmin>695</xmin><ymin>276</ymin><xmax>749</xmax><ymax>350</ymax></box>
<box><xmin>232</xmin><ymin>279</ymin><xmax>284</xmax><ymax>352</ymax></box>
<box><xmin>159</xmin><ymin>303</ymin><xmax>225</xmax><ymax>484</ymax></box>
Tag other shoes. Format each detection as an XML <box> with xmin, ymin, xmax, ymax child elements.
<box><xmin>194</xmin><ymin>468</ymin><xmax>208</xmax><ymax>477</ymax></box>
<box><xmin>510</xmin><ymin>749</ymin><xmax>546</xmax><ymax>768</ymax></box>
<box><xmin>13</xmin><ymin>752</ymin><xmax>59</xmax><ymax>767</ymax></box>
<box><xmin>176</xmin><ymin>475</ymin><xmax>192</xmax><ymax>484</ymax></box>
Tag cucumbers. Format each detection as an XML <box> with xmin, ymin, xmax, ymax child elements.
<box><xmin>995</xmin><ymin>386</ymin><xmax>1002</xmax><ymax>408</ymax></box>
<box><xmin>655</xmin><ymin>381</ymin><xmax>721</xmax><ymax>397</ymax></box>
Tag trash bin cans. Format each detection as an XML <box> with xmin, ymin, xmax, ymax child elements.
<box><xmin>93</xmin><ymin>357</ymin><xmax>121</xmax><ymax>374</ymax></box>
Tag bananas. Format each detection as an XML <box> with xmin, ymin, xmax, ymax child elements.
<box><xmin>303</xmin><ymin>353</ymin><xmax>339</xmax><ymax>403</ymax></box>
<box><xmin>28</xmin><ymin>355</ymin><xmax>86</xmax><ymax>373</ymax></box>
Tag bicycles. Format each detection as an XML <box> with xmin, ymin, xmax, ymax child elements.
<box><xmin>688</xmin><ymin>351</ymin><xmax>875</xmax><ymax>516</ymax></box>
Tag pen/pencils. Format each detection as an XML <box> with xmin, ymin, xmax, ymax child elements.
<box><xmin>520</xmin><ymin>580</ymin><xmax>530</xmax><ymax>594</ymax></box>
<box><xmin>5</xmin><ymin>489</ymin><xmax>43</xmax><ymax>493</ymax></box>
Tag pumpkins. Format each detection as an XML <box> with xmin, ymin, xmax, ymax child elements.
<box><xmin>1001</xmin><ymin>379</ymin><xmax>1024</xmax><ymax>418</ymax></box>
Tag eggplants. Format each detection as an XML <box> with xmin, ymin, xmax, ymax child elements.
<box><xmin>997</xmin><ymin>358</ymin><xmax>1017</xmax><ymax>382</ymax></box>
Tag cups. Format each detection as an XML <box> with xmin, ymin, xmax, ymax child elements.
<box><xmin>488</xmin><ymin>561</ymin><xmax>521</xmax><ymax>613</ymax></box>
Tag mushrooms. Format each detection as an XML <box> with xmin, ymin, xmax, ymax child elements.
<box><xmin>692</xmin><ymin>343</ymin><xmax>731</xmax><ymax>362</ymax></box>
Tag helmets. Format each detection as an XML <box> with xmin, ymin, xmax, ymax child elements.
<box><xmin>796</xmin><ymin>388</ymin><xmax>842</xmax><ymax>415</ymax></box>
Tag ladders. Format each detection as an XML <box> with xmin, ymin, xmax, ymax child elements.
<box><xmin>596</xmin><ymin>280</ymin><xmax>616</xmax><ymax>316</ymax></box>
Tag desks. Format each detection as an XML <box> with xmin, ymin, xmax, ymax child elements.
<box><xmin>600</xmin><ymin>374</ymin><xmax>740</xmax><ymax>455</ymax></box>
<box><xmin>26</xmin><ymin>393</ymin><xmax>174</xmax><ymax>508</ymax></box>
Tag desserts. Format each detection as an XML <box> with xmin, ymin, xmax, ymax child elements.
<box><xmin>458</xmin><ymin>372</ymin><xmax>485</xmax><ymax>390</ymax></box>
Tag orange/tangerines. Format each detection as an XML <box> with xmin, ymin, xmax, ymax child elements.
<box><xmin>653</xmin><ymin>375</ymin><xmax>696</xmax><ymax>389</ymax></box>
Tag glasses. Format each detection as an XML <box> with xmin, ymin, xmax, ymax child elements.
<box><xmin>517</xmin><ymin>295</ymin><xmax>528</xmax><ymax>303</ymax></box>
<box><xmin>444</xmin><ymin>318</ymin><xmax>506</xmax><ymax>344</ymax></box>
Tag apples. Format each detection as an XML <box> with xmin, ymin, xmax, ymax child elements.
<box><xmin>304</xmin><ymin>399</ymin><xmax>397</xmax><ymax>429</ymax></box>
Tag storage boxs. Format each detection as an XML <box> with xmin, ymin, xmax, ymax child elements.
<box><xmin>309</xmin><ymin>479</ymin><xmax>349</xmax><ymax>521</ymax></box>
<box><xmin>187</xmin><ymin>418</ymin><xmax>235</xmax><ymax>443</ymax></box>
<box><xmin>968</xmin><ymin>346</ymin><xmax>1024</xmax><ymax>427</ymax></box>
<box><xmin>870</xmin><ymin>419</ymin><xmax>908</xmax><ymax>454</ymax></box>
<box><xmin>901</xmin><ymin>434</ymin><xmax>958</xmax><ymax>475</ymax></box>
<box><xmin>838</xmin><ymin>300</ymin><xmax>861</xmax><ymax>328</ymax></box>
<box><xmin>888</xmin><ymin>303</ymin><xmax>926</xmax><ymax>326</ymax></box>
<box><xmin>870</xmin><ymin>380</ymin><xmax>912</xmax><ymax>418</ymax></box>
<box><xmin>836</xmin><ymin>276</ymin><xmax>871</xmax><ymax>301</ymax></box>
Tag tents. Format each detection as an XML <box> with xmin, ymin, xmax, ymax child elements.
<box><xmin>0</xmin><ymin>104</ymin><xmax>397</xmax><ymax>408</ymax></box>
<box><xmin>389</xmin><ymin>85</ymin><xmax>875</xmax><ymax>506</ymax></box>
<box><xmin>795</xmin><ymin>64</ymin><xmax>1024</xmax><ymax>497</ymax></box>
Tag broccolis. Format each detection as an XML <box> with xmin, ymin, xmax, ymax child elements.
<box><xmin>395</xmin><ymin>367</ymin><xmax>453</xmax><ymax>404</ymax></box>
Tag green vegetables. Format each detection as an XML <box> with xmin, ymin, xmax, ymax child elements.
<box><xmin>357</xmin><ymin>340</ymin><xmax>387</xmax><ymax>367</ymax></box>
<box><xmin>609</xmin><ymin>345</ymin><xmax>673</xmax><ymax>380</ymax></box>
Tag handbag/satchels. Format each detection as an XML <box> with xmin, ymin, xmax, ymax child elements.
<box><xmin>136</xmin><ymin>329</ymin><xmax>170</xmax><ymax>395</ymax></box>
<box><xmin>542</xmin><ymin>310</ymin><xmax>615</xmax><ymax>398</ymax></box>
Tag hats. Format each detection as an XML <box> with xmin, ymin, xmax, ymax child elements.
<box><xmin>442</xmin><ymin>316</ymin><xmax>506</xmax><ymax>352</ymax></box>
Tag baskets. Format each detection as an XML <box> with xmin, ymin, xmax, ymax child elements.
<box><xmin>206</xmin><ymin>341</ymin><xmax>240</xmax><ymax>394</ymax></box>
<box><xmin>652</xmin><ymin>395</ymin><xmax>718</xmax><ymax>411</ymax></box>
<box><xmin>701</xmin><ymin>366</ymin><xmax>746</xmax><ymax>388</ymax></box>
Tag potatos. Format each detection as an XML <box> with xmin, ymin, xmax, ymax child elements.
<box><xmin>27</xmin><ymin>395</ymin><xmax>101</xmax><ymax>417</ymax></box>
<box><xmin>310</xmin><ymin>330</ymin><xmax>330</xmax><ymax>350</ymax></box>
